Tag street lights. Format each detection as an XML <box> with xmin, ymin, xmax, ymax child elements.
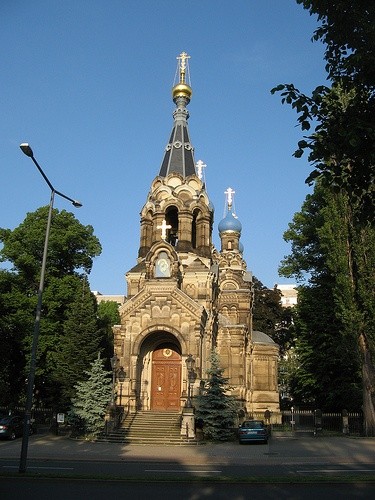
<box><xmin>110</xmin><ymin>351</ymin><xmax>120</xmax><ymax>410</ymax></box>
<box><xmin>18</xmin><ymin>143</ymin><xmax>83</xmax><ymax>474</ymax></box>
<box><xmin>184</xmin><ymin>353</ymin><xmax>195</xmax><ymax>409</ymax></box>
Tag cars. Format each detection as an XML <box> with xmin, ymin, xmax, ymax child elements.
<box><xmin>0</xmin><ymin>415</ymin><xmax>37</xmax><ymax>440</ymax></box>
<box><xmin>238</xmin><ymin>419</ymin><xmax>270</xmax><ymax>444</ymax></box>
